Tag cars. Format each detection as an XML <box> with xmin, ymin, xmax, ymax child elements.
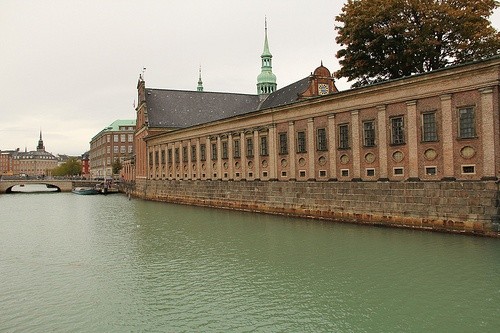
<box><xmin>94</xmin><ymin>176</ymin><xmax>112</xmax><ymax>182</ymax></box>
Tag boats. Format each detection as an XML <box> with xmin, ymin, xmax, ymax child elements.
<box><xmin>71</xmin><ymin>181</ymin><xmax>120</xmax><ymax>195</ymax></box>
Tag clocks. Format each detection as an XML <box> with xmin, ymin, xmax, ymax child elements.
<box><xmin>317</xmin><ymin>83</ymin><xmax>329</xmax><ymax>95</ymax></box>
